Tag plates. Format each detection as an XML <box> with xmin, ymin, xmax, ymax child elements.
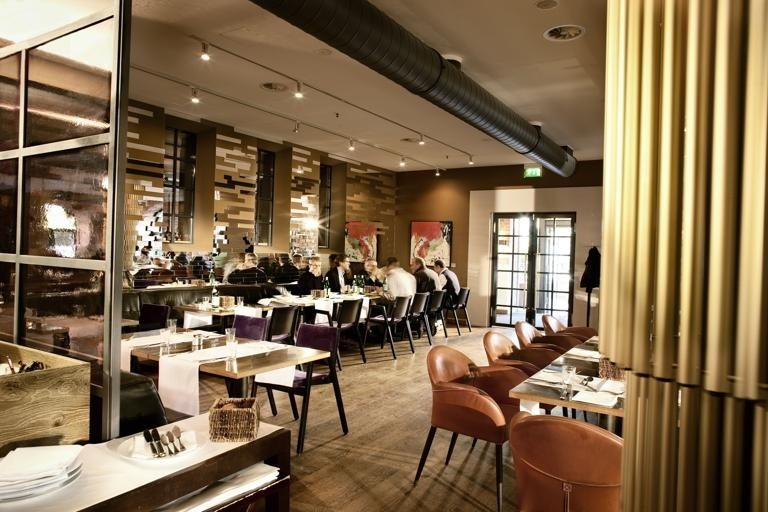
<box><xmin>0</xmin><ymin>456</ymin><xmax>82</xmax><ymax>506</ymax></box>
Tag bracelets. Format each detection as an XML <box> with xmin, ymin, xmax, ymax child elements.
<box><xmin>346</xmin><ymin>272</ymin><xmax>351</xmax><ymax>275</ymax></box>
<box><xmin>372</xmin><ymin>273</ymin><xmax>376</xmax><ymax>277</ymax></box>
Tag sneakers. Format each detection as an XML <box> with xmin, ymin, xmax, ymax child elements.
<box><xmin>378</xmin><ymin>326</ymin><xmax>387</xmax><ymax>349</ymax></box>
<box><xmin>416</xmin><ymin>320</ymin><xmax>424</xmax><ymax>338</ymax></box>
<box><xmin>429</xmin><ymin>320</ymin><xmax>437</xmax><ymax>337</ymax></box>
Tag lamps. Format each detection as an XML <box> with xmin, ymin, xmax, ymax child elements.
<box><xmin>293</xmin><ymin>120</ymin><xmax>302</xmax><ymax>135</ymax></box>
<box><xmin>435</xmin><ymin>167</ymin><xmax>441</xmax><ymax>176</ymax></box>
<box><xmin>295</xmin><ymin>81</ymin><xmax>304</xmax><ymax>98</ymax></box>
<box><xmin>400</xmin><ymin>157</ymin><xmax>405</xmax><ymax>167</ymax></box>
<box><xmin>468</xmin><ymin>156</ymin><xmax>474</xmax><ymax>165</ymax></box>
<box><xmin>349</xmin><ymin>138</ymin><xmax>355</xmax><ymax>151</ymax></box>
<box><xmin>418</xmin><ymin>134</ymin><xmax>425</xmax><ymax>145</ymax></box>
<box><xmin>200</xmin><ymin>41</ymin><xmax>213</xmax><ymax>61</ymax></box>
<box><xmin>192</xmin><ymin>88</ymin><xmax>201</xmax><ymax>104</ymax></box>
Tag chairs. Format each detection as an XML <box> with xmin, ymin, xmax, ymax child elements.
<box><xmin>126</xmin><ymin>303</ymin><xmax>169</xmax><ymax>331</ymax></box>
<box><xmin>509</xmin><ymin>415</ymin><xmax>624</xmax><ymax>512</ymax></box>
<box><xmin>360</xmin><ymin>295</ymin><xmax>414</xmax><ymax>359</ymax></box>
<box><xmin>252</xmin><ymin>323</ymin><xmax>349</xmax><ymax>453</ymax></box>
<box><xmin>483</xmin><ymin>331</ymin><xmax>562</xmax><ymax>376</ymax></box>
<box><xmin>516</xmin><ymin>321</ymin><xmax>583</xmax><ymax>354</ymax></box>
<box><xmin>542</xmin><ymin>315</ymin><xmax>598</xmax><ymax>335</ymax></box>
<box><xmin>447</xmin><ymin>287</ymin><xmax>472</xmax><ymax>337</ymax></box>
<box><xmin>413</xmin><ymin>290</ymin><xmax>447</xmax><ymax>345</ymax></box>
<box><xmin>413</xmin><ymin>345</ymin><xmax>537</xmax><ymax>511</ymax></box>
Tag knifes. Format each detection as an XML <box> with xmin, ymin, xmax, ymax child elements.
<box><xmin>142</xmin><ymin>429</ymin><xmax>158</xmax><ymax>457</ymax></box>
<box><xmin>152</xmin><ymin>427</ymin><xmax>166</xmax><ymax>458</ymax></box>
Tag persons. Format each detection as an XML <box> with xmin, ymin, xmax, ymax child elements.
<box><xmin>274</xmin><ymin>252</ymin><xmax>299</xmax><ymax>283</ymax></box>
<box><xmin>329</xmin><ymin>254</ymin><xmax>353</xmax><ymax>279</ymax></box>
<box><xmin>229</xmin><ymin>252</ymin><xmax>268</xmax><ymax>305</ymax></box>
<box><xmin>288</xmin><ymin>250</ymin><xmax>307</xmax><ymax>270</ymax></box>
<box><xmin>324</xmin><ymin>253</ymin><xmax>354</xmax><ymax>293</ymax></box>
<box><xmin>385</xmin><ymin>257</ymin><xmax>418</xmax><ymax>315</ymax></box>
<box><xmin>410</xmin><ymin>256</ymin><xmax>441</xmax><ymax>314</ymax></box>
<box><xmin>434</xmin><ymin>259</ymin><xmax>461</xmax><ymax>310</ymax></box>
<box><xmin>356</xmin><ymin>258</ymin><xmax>384</xmax><ymax>287</ymax></box>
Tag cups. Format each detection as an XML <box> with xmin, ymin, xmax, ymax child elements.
<box><xmin>177</xmin><ymin>279</ymin><xmax>205</xmax><ymax>285</ymax></box>
<box><xmin>200</xmin><ymin>293</ymin><xmax>244</xmax><ymax>309</ymax></box>
<box><xmin>225</xmin><ymin>361</ymin><xmax>238</xmax><ymax>373</ymax></box>
<box><xmin>159</xmin><ymin>316</ymin><xmax>238</xmax><ymax>361</ymax></box>
<box><xmin>562</xmin><ymin>366</ymin><xmax>577</xmax><ymax>385</ymax></box>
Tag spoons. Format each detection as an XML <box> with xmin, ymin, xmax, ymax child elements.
<box><xmin>583</xmin><ymin>376</ymin><xmax>593</xmax><ymax>386</ymax></box>
<box><xmin>161</xmin><ymin>425</ymin><xmax>185</xmax><ymax>455</ymax></box>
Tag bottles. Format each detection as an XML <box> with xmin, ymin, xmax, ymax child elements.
<box><xmin>163</xmin><ymin>227</ymin><xmax>182</xmax><ymax>242</ymax></box>
<box><xmin>308</xmin><ymin>272</ymin><xmax>388</xmax><ymax>299</ymax></box>
<box><xmin>209</xmin><ymin>271</ymin><xmax>215</xmax><ymax>284</ymax></box>
